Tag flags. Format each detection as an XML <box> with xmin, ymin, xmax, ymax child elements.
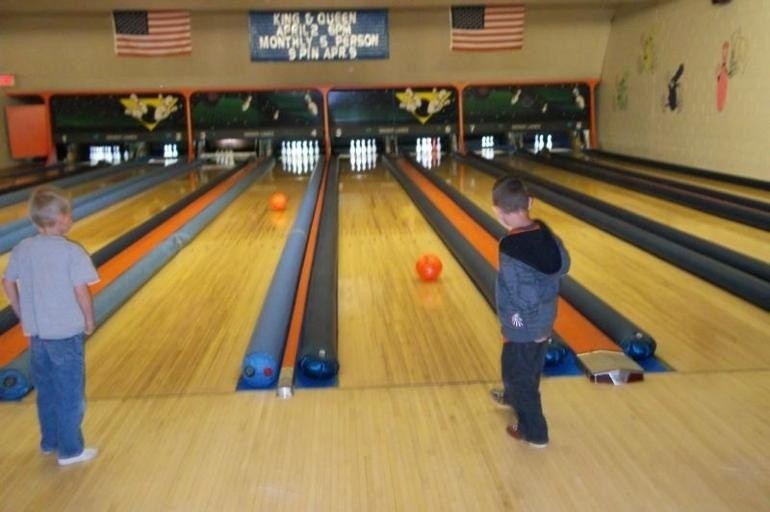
<box><xmin>107</xmin><ymin>7</ymin><xmax>192</xmax><ymax>58</ymax></box>
<box><xmin>448</xmin><ymin>2</ymin><xmax>526</xmax><ymax>52</ymax></box>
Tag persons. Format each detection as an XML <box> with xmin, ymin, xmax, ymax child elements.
<box><xmin>489</xmin><ymin>174</ymin><xmax>572</xmax><ymax>446</ymax></box>
<box><xmin>1</xmin><ymin>183</ymin><xmax>103</xmax><ymax>467</ymax></box>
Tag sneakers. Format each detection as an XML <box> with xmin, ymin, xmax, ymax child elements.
<box><xmin>506</xmin><ymin>420</ymin><xmax>549</xmax><ymax>448</ymax></box>
<box><xmin>488</xmin><ymin>385</ymin><xmax>521</xmax><ymax>412</ymax></box>
